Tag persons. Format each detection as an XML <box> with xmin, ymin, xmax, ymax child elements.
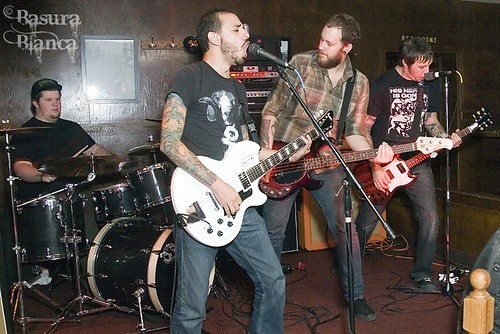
<box><xmin>260</xmin><ymin>15</ymin><xmax>395</xmax><ymax>323</ymax></box>
<box><xmin>329</xmin><ymin>38</ymin><xmax>462</xmax><ymax>291</ymax></box>
<box><xmin>7</xmin><ymin>78</ymin><xmax>111</xmax><ymax>288</ymax></box>
<box><xmin>160</xmin><ymin>9</ymin><xmax>286</xmax><ymax>334</ymax></box>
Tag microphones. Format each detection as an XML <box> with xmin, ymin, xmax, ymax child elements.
<box><xmin>248</xmin><ymin>43</ymin><xmax>296</xmax><ymax>71</ymax></box>
<box><xmin>424</xmin><ymin>70</ymin><xmax>457</xmax><ymax>81</ymax></box>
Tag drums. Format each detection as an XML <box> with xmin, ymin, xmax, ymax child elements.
<box><xmin>15</xmin><ymin>194</ymin><xmax>92</xmax><ymax>264</ymax></box>
<box><xmin>90</xmin><ymin>183</ymin><xmax>138</xmax><ymax>228</ymax></box>
<box><xmin>85</xmin><ymin>216</ymin><xmax>216</xmax><ymax>317</ymax></box>
<box><xmin>124</xmin><ymin>163</ymin><xmax>172</xmax><ymax>211</ymax></box>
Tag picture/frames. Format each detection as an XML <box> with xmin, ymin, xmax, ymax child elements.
<box><xmin>80</xmin><ymin>34</ymin><xmax>140</xmax><ymax>104</ymax></box>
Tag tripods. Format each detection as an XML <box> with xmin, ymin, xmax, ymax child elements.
<box><xmin>404</xmin><ymin>77</ymin><xmax>464</xmax><ymax>309</ymax></box>
<box><xmin>5</xmin><ymin>132</ymin><xmax>142</xmax><ymax>334</ymax></box>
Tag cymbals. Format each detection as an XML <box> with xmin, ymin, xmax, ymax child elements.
<box><xmin>145</xmin><ymin>118</ymin><xmax>162</xmax><ymax>123</ymax></box>
<box><xmin>127</xmin><ymin>142</ymin><xmax>160</xmax><ymax>152</ymax></box>
<box><xmin>40</xmin><ymin>155</ymin><xmax>130</xmax><ymax>178</ymax></box>
<box><xmin>0</xmin><ymin>123</ymin><xmax>55</xmax><ymax>134</ymax></box>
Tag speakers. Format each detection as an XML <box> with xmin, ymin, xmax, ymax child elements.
<box><xmin>282</xmin><ymin>187</ymin><xmax>386</xmax><ymax>254</ymax></box>
<box><xmin>457</xmin><ymin>228</ymin><xmax>500</xmax><ymax>334</ymax></box>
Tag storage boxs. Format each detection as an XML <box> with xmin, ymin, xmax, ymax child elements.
<box><xmin>281</xmin><ymin>185</ymin><xmax>387</xmax><ymax>253</ymax></box>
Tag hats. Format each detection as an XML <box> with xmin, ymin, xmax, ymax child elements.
<box><xmin>31</xmin><ymin>79</ymin><xmax>62</xmax><ymax>97</ymax></box>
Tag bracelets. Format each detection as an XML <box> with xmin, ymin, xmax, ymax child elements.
<box><xmin>40</xmin><ymin>173</ymin><xmax>45</xmax><ymax>182</ymax></box>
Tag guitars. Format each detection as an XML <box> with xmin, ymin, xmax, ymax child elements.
<box><xmin>340</xmin><ymin>107</ymin><xmax>496</xmax><ymax>206</ymax></box>
<box><xmin>258</xmin><ymin>136</ymin><xmax>454</xmax><ymax>201</ymax></box>
<box><xmin>169</xmin><ymin>108</ymin><xmax>334</xmax><ymax>247</ymax></box>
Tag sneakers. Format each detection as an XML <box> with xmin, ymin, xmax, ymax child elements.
<box><xmin>417</xmin><ymin>277</ymin><xmax>436</xmax><ymax>290</ymax></box>
<box><xmin>353</xmin><ymin>298</ymin><xmax>376</xmax><ymax>321</ymax></box>
